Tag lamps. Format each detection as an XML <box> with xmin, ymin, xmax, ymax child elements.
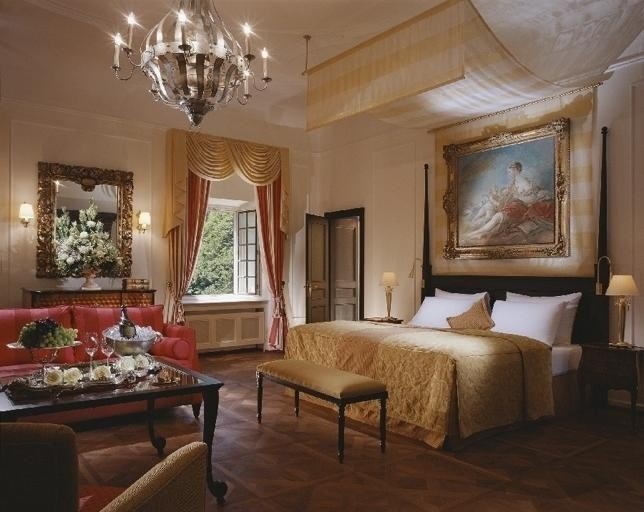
<box><xmin>606</xmin><ymin>275</ymin><xmax>639</xmax><ymax>348</ymax></box>
<box><xmin>138</xmin><ymin>211</ymin><xmax>152</xmax><ymax>234</ymax></box>
<box><xmin>112</xmin><ymin>0</ymin><xmax>274</xmax><ymax>126</ymax></box>
<box><xmin>379</xmin><ymin>271</ymin><xmax>401</xmax><ymax>321</ymax></box>
<box><xmin>18</xmin><ymin>202</ymin><xmax>34</xmax><ymax>227</ymax></box>
<box><xmin>407</xmin><ymin>258</ymin><xmax>423</xmax><ymax>279</ymax></box>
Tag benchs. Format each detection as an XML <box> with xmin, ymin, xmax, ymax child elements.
<box><xmin>256</xmin><ymin>359</ymin><xmax>387</xmax><ymax>464</ymax></box>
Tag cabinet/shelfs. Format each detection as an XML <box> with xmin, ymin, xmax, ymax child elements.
<box><xmin>177</xmin><ymin>310</ymin><xmax>264</xmax><ymax>351</ymax></box>
<box><xmin>21</xmin><ymin>288</ymin><xmax>156</xmax><ymax>308</ymax></box>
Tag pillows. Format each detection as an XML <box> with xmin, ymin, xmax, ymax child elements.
<box><xmin>0</xmin><ymin>304</ymin><xmax>164</xmax><ymax>366</ymax></box>
<box><xmin>409</xmin><ymin>288</ymin><xmax>494</xmax><ymax>346</ymax></box>
<box><xmin>490</xmin><ymin>291</ymin><xmax>583</xmax><ymax>346</ymax></box>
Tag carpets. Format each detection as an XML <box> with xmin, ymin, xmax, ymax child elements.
<box><xmin>496</xmin><ymin>420</ymin><xmax>609</xmax><ymax>460</ymax></box>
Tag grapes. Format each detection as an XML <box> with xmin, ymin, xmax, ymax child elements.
<box><xmin>16</xmin><ymin>316</ymin><xmax>78</xmax><ymax>347</ymax></box>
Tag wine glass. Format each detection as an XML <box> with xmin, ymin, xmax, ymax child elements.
<box><xmin>83</xmin><ymin>330</ymin><xmax>100</xmax><ymax>374</ymax></box>
<box><xmin>101</xmin><ymin>329</ymin><xmax>118</xmax><ymax>367</ymax></box>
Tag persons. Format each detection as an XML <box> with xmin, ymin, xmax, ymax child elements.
<box><xmin>468</xmin><ymin>161</ymin><xmax>536</xmax><ymax>240</ymax></box>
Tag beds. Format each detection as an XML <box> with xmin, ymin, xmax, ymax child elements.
<box><xmin>284</xmin><ymin>320</ymin><xmax>583</xmax><ymax>452</ymax></box>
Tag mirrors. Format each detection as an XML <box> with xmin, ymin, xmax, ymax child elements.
<box><xmin>37</xmin><ymin>160</ymin><xmax>134</xmax><ymax>279</ymax></box>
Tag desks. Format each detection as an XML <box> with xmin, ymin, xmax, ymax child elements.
<box><xmin>0</xmin><ymin>357</ymin><xmax>228</xmax><ymax>499</ymax></box>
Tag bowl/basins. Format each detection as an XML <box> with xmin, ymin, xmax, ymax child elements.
<box><xmin>101</xmin><ymin>329</ymin><xmax>163</xmax><ymax>359</ymax></box>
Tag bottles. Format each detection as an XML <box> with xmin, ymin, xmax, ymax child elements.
<box><xmin>118</xmin><ymin>303</ymin><xmax>137</xmax><ymax>339</ymax></box>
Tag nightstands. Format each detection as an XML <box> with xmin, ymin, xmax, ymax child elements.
<box><xmin>582</xmin><ymin>343</ymin><xmax>644</xmax><ymax>432</ymax></box>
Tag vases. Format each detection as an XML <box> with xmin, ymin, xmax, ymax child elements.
<box><xmin>80</xmin><ymin>268</ymin><xmax>102</xmax><ymax>290</ymax></box>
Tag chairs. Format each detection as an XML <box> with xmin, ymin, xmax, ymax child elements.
<box><xmin>1</xmin><ymin>422</ymin><xmax>209</xmax><ymax>512</ymax></box>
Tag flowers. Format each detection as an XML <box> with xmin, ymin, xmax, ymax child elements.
<box><xmin>57</xmin><ymin>199</ymin><xmax>123</xmax><ymax>279</ymax></box>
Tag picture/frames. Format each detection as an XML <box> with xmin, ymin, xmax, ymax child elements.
<box><xmin>442</xmin><ymin>118</ymin><xmax>571</xmax><ymax>260</ymax></box>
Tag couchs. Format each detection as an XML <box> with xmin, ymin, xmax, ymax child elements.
<box><xmin>0</xmin><ymin>304</ymin><xmax>202</xmax><ymax>426</ymax></box>
